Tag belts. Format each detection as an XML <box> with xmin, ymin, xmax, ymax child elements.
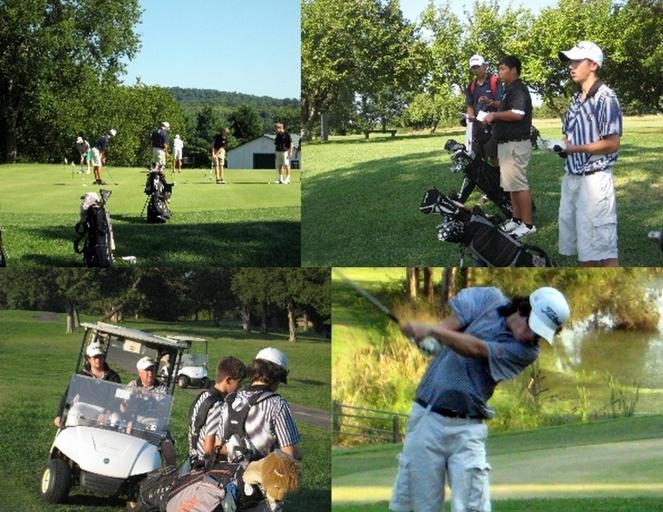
<box><xmin>414</xmin><ymin>398</ymin><xmax>485</xmax><ymax>419</ymax></box>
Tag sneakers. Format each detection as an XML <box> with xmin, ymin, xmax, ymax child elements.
<box><xmin>279</xmin><ymin>180</ymin><xmax>291</xmax><ymax>184</ymax></box>
<box><xmin>500</xmin><ymin>218</ymin><xmax>520</xmax><ymax>232</ymax></box>
<box><xmin>216</xmin><ymin>180</ymin><xmax>225</xmax><ymax>184</ymax></box>
<box><xmin>510</xmin><ymin>223</ymin><xmax>537</xmax><ymax>239</ymax></box>
<box><xmin>95</xmin><ymin>180</ymin><xmax>107</xmax><ymax>185</ymax></box>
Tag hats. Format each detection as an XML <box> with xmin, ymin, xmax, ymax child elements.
<box><xmin>136</xmin><ymin>356</ymin><xmax>155</xmax><ymax>370</ymax></box>
<box><xmin>528</xmin><ymin>287</ymin><xmax>569</xmax><ymax>345</ymax></box>
<box><xmin>86</xmin><ymin>342</ymin><xmax>105</xmax><ymax>357</ymax></box>
<box><xmin>255</xmin><ymin>348</ymin><xmax>288</xmax><ymax>371</ymax></box>
<box><xmin>162</xmin><ymin>121</ymin><xmax>170</xmax><ymax>127</ymax></box>
<box><xmin>558</xmin><ymin>41</ymin><xmax>603</xmax><ymax>68</ymax></box>
<box><xmin>469</xmin><ymin>55</ymin><xmax>484</xmax><ymax>68</ymax></box>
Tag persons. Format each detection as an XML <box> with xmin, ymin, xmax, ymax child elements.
<box><xmin>385</xmin><ymin>278</ymin><xmax>569</xmax><ymax>512</ymax></box>
<box><xmin>458</xmin><ymin>53</ymin><xmax>541</xmax><ymax>241</ymax></box>
<box><xmin>198</xmin><ymin>346</ymin><xmax>301</xmax><ymax>510</ymax></box>
<box><xmin>53</xmin><ymin>342</ymin><xmax>121</xmax><ymax>429</ymax></box>
<box><xmin>117</xmin><ymin>352</ymin><xmax>170</xmax><ymax>432</ymax></box>
<box><xmin>75</xmin><ymin>120</ymin><xmax>294</xmax><ymax>186</ymax></box>
<box><xmin>181</xmin><ymin>356</ymin><xmax>247</xmax><ymax>462</ymax></box>
<box><xmin>547</xmin><ymin>38</ymin><xmax>623</xmax><ymax>267</ymax></box>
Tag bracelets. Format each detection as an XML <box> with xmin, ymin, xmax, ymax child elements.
<box><xmin>486</xmin><ymin>99</ymin><xmax>495</xmax><ymax>107</ymax></box>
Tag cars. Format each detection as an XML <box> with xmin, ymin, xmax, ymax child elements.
<box><xmin>161</xmin><ymin>333</ymin><xmax>212</xmax><ymax>387</ymax></box>
<box><xmin>36</xmin><ymin>318</ymin><xmax>184</xmax><ymax>504</ymax></box>
